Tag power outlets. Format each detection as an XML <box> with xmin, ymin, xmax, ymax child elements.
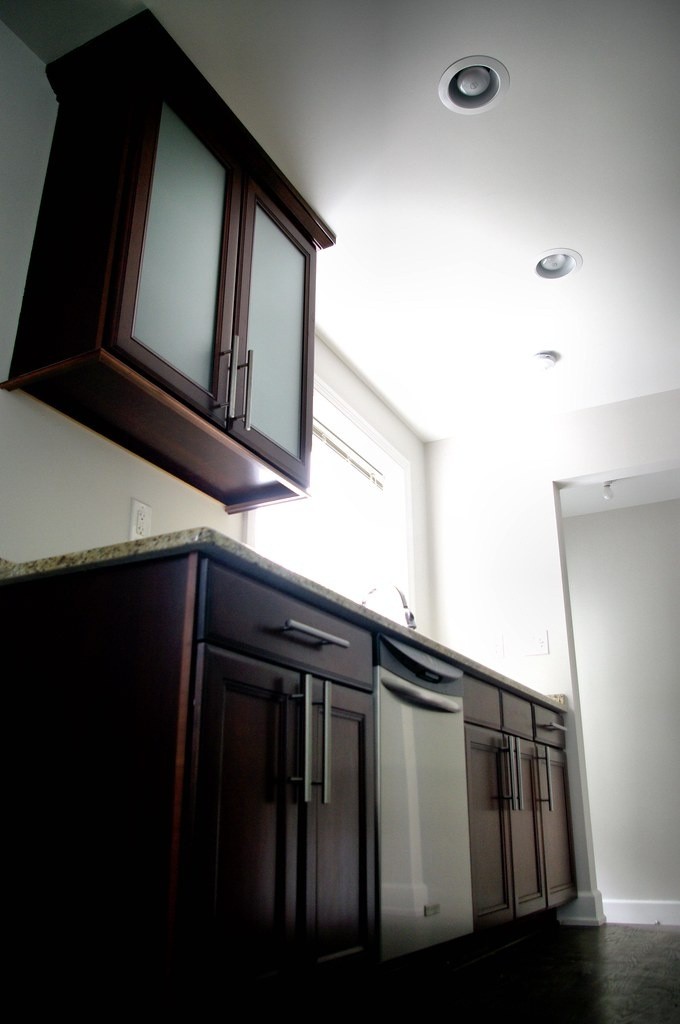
<box><xmin>129</xmin><ymin>498</ymin><xmax>153</xmax><ymax>540</ymax></box>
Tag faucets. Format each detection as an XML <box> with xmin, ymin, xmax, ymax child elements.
<box><xmin>361</xmin><ymin>581</ymin><xmax>416</xmax><ymax>629</ymax></box>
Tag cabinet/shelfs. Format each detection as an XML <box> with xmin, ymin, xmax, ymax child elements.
<box><xmin>180</xmin><ymin>554</ymin><xmax>380</xmax><ymax>1024</ymax></box>
<box><xmin>0</xmin><ymin>7</ymin><xmax>334</xmax><ymax>514</ymax></box>
<box><xmin>462</xmin><ymin>674</ymin><xmax>579</xmax><ymax>928</ymax></box>
